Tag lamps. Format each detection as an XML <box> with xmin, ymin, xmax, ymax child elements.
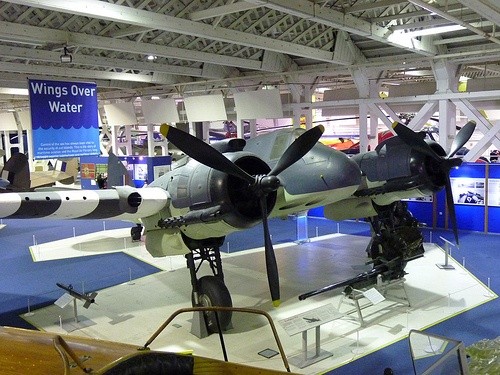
<box><xmin>61</xmin><ymin>47</ymin><xmax>74</xmax><ymax>62</ymax></box>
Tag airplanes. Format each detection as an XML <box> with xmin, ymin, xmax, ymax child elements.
<box><xmin>0</xmin><ymin>121</ymin><xmax>477</xmax><ymax>333</ymax></box>
